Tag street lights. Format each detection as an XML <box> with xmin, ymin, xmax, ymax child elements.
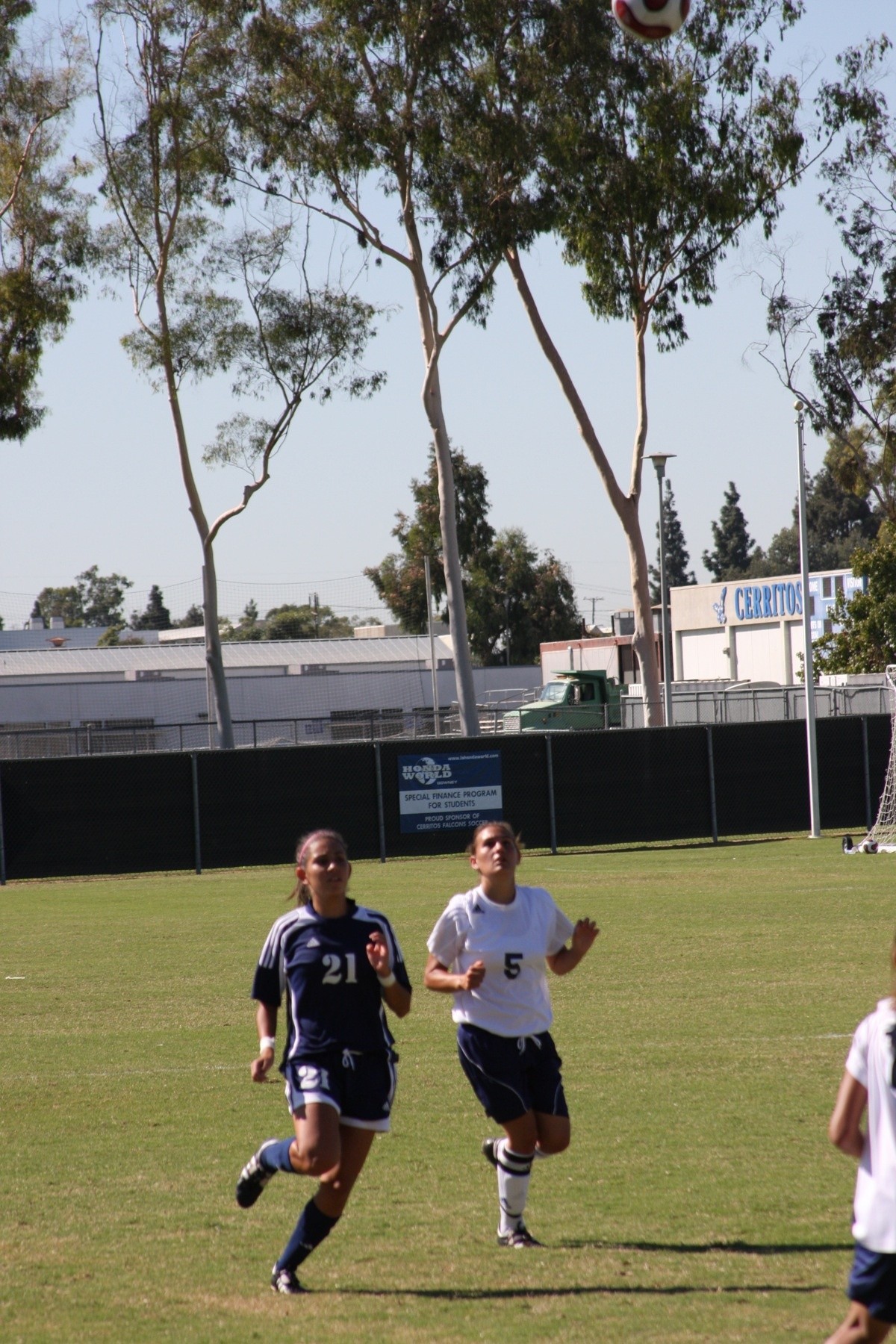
<box><xmin>640</xmin><ymin>451</ymin><xmax>677</xmax><ymax>726</ymax></box>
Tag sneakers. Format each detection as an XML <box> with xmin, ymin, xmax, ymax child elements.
<box><xmin>495</xmin><ymin>1220</ymin><xmax>546</xmax><ymax>1250</ymax></box>
<box><xmin>270</xmin><ymin>1263</ymin><xmax>309</xmax><ymax>1294</ymax></box>
<box><xmin>481</xmin><ymin>1137</ymin><xmax>500</xmax><ymax>1170</ymax></box>
<box><xmin>236</xmin><ymin>1138</ymin><xmax>283</xmax><ymax>1209</ymax></box>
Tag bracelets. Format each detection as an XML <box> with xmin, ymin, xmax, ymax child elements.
<box><xmin>259</xmin><ymin>1037</ymin><xmax>274</xmax><ymax>1052</ymax></box>
<box><xmin>378</xmin><ymin>972</ymin><xmax>396</xmax><ymax>988</ymax></box>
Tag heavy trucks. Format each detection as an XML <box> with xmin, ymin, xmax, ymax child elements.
<box><xmin>502</xmin><ymin>669</ymin><xmax>890</xmax><ymax>734</ymax></box>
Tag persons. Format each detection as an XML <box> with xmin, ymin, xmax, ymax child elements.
<box><xmin>819</xmin><ymin>939</ymin><xmax>896</xmax><ymax>1344</ymax></box>
<box><xmin>424</xmin><ymin>818</ymin><xmax>606</xmax><ymax>1252</ymax></box>
<box><xmin>236</xmin><ymin>829</ymin><xmax>411</xmax><ymax>1293</ymax></box>
